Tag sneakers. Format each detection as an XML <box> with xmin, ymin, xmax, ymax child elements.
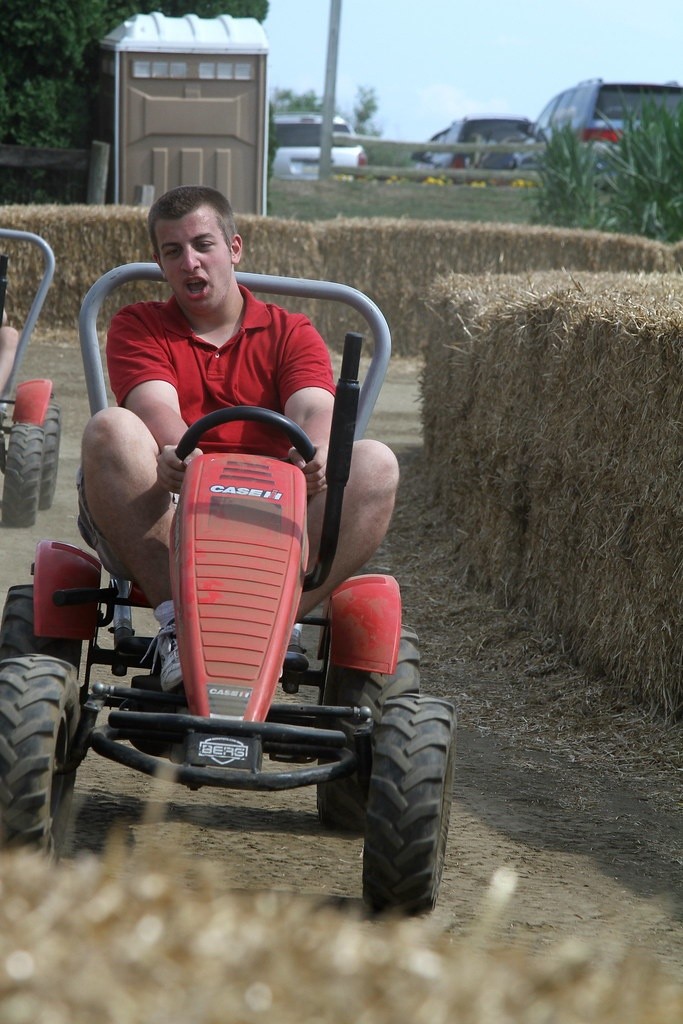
<box><xmin>285</xmin><ymin>643</ymin><xmax>309</xmax><ymax>673</ymax></box>
<box><xmin>139</xmin><ymin>618</ymin><xmax>183</xmax><ymax>690</ymax></box>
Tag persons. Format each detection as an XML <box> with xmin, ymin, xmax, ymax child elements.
<box><xmin>0</xmin><ymin>307</ymin><xmax>20</xmax><ymax>398</ymax></box>
<box><xmin>78</xmin><ymin>185</ymin><xmax>400</xmax><ymax>693</ymax></box>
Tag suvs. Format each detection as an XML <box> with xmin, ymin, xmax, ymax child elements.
<box><xmin>412</xmin><ymin>116</ymin><xmax>534</xmax><ymax>185</ymax></box>
<box><xmin>510</xmin><ymin>77</ymin><xmax>682</xmax><ymax>189</ymax></box>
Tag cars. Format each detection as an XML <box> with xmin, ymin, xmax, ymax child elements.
<box><xmin>268</xmin><ymin>112</ymin><xmax>368</xmax><ymax>182</ymax></box>
